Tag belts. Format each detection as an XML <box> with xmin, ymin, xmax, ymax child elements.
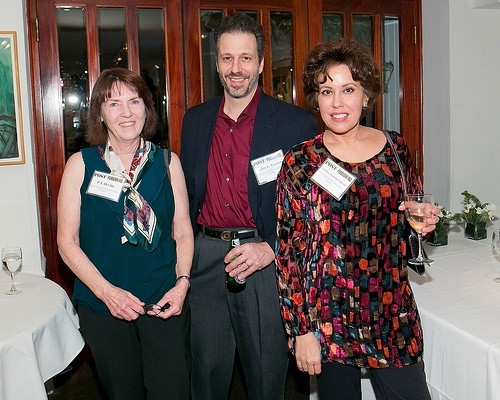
<box><xmin>198</xmin><ymin>224</ymin><xmax>258</xmax><ymax>241</ymax></box>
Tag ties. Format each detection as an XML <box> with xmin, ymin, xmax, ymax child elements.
<box><xmin>98</xmin><ymin>134</ymin><xmax>162</xmax><ymax>254</ymax></box>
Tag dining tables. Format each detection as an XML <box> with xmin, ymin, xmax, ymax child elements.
<box><xmin>408</xmin><ymin>214</ymin><xmax>500</xmax><ymax>400</ymax></box>
<box><xmin>0</xmin><ymin>270</ymin><xmax>84</xmax><ymax>400</ymax></box>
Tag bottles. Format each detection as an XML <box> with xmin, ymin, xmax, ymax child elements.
<box><xmin>225</xmin><ymin>231</ymin><xmax>246</xmax><ymax>293</ymax></box>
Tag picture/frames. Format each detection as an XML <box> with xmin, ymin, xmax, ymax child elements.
<box><xmin>0</xmin><ymin>30</ymin><xmax>27</xmax><ymax>165</ymax></box>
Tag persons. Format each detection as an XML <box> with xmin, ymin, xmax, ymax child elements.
<box><xmin>58</xmin><ymin>70</ymin><xmax>171</xmax><ymax>158</ymax></box>
<box><xmin>274</xmin><ymin>37</ymin><xmax>441</xmax><ymax>400</ymax></box>
<box><xmin>57</xmin><ymin>67</ymin><xmax>195</xmax><ymax>400</ymax></box>
<box><xmin>176</xmin><ymin>12</ymin><xmax>318</xmax><ymax>400</ymax></box>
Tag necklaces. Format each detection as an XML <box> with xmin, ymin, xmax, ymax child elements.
<box><xmin>115</xmin><ymin>151</ymin><xmax>136</xmax><ymax>158</ymax></box>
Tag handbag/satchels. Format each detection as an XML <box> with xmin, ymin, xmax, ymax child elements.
<box><xmin>380</xmin><ymin>129</ymin><xmax>431</xmax><ymax>275</ymax></box>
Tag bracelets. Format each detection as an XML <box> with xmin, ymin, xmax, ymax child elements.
<box><xmin>176</xmin><ymin>275</ymin><xmax>192</xmax><ymax>289</ymax></box>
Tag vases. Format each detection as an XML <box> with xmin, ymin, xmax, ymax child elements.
<box><xmin>462</xmin><ymin>221</ymin><xmax>487</xmax><ymax>240</ymax></box>
<box><xmin>426</xmin><ymin>228</ymin><xmax>448</xmax><ymax>247</ymax></box>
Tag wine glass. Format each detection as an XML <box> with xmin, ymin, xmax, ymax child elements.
<box><xmin>492</xmin><ymin>231</ymin><xmax>500</xmax><ymax>283</ymax></box>
<box><xmin>4</xmin><ymin>247</ymin><xmax>22</xmax><ymax>294</ymax></box>
<box><xmin>404</xmin><ymin>194</ymin><xmax>434</xmax><ymax>265</ymax></box>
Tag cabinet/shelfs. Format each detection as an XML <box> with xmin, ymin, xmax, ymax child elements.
<box><xmin>25</xmin><ymin>0</ymin><xmax>423</xmax><ymax>300</ymax></box>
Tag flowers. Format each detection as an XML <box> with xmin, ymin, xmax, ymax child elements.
<box><xmin>450</xmin><ymin>190</ymin><xmax>497</xmax><ymax>224</ymax></box>
<box><xmin>433</xmin><ymin>202</ymin><xmax>454</xmax><ymax>227</ymax></box>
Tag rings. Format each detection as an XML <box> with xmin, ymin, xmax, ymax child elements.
<box><xmin>244</xmin><ymin>262</ymin><xmax>250</xmax><ymax>269</ymax></box>
